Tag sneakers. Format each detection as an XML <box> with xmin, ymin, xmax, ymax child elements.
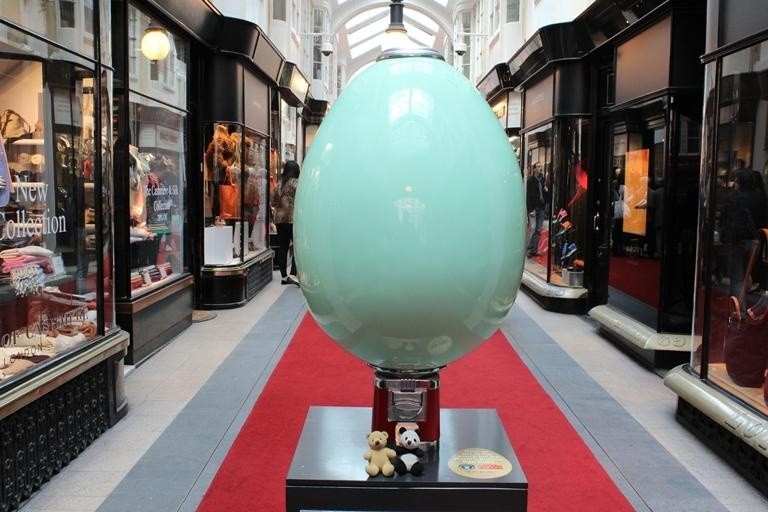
<box><xmin>551</xmin><ymin>206</ymin><xmax>582</xmax><ymax>261</ymax></box>
<box><xmin>635</xmin><ymin>187</ymin><xmax>664</xmax><ymax>209</ymax></box>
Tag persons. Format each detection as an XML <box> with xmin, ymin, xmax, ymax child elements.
<box><xmin>124</xmin><ymin>144</ymin><xmax>153</xmax><ymax>268</ymax></box>
<box><xmin>525</xmin><ymin>161</ymin><xmax>545</xmax><ymax>259</ymax></box>
<box><xmin>717</xmin><ymin>168</ymin><xmax>768</xmax><ymax>323</ymax></box>
<box><xmin>277</xmin><ymin>161</ymin><xmax>302</xmax><ymax>288</ymax></box>
<box><xmin>205</xmin><ymin>124</ymin><xmax>235</xmax><ymax>228</ymax></box>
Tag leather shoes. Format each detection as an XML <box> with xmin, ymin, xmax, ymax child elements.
<box><xmin>281</xmin><ymin>276</ymin><xmax>300</xmax><ymax>286</ymax></box>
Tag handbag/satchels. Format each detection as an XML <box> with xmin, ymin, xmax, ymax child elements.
<box><xmin>611</xmin><ymin>199</ymin><xmax>632</xmax><ymax>221</ymax></box>
<box><xmin>218</xmin><ymin>167</ymin><xmax>242</xmax><ymax>220</ymax></box>
<box><xmin>722</xmin><ymin>227</ymin><xmax>768</xmax><ymax>388</ymax></box>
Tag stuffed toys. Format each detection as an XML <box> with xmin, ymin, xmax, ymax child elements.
<box><xmin>364</xmin><ymin>429</ymin><xmax>396</xmax><ymax>476</ymax></box>
<box><xmin>396</xmin><ymin>426</ymin><xmax>425</xmax><ymax>474</ymax></box>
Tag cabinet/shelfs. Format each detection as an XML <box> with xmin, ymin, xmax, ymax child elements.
<box><xmin>2</xmin><ymin>137</ymin><xmax>48</xmax><ymax>232</ymax></box>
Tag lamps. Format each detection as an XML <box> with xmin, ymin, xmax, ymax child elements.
<box><xmin>379</xmin><ymin>1</ymin><xmax>409</xmax><ymax>51</ymax></box>
<box><xmin>142</xmin><ymin>18</ymin><xmax>171</xmax><ymax>64</ymax></box>
